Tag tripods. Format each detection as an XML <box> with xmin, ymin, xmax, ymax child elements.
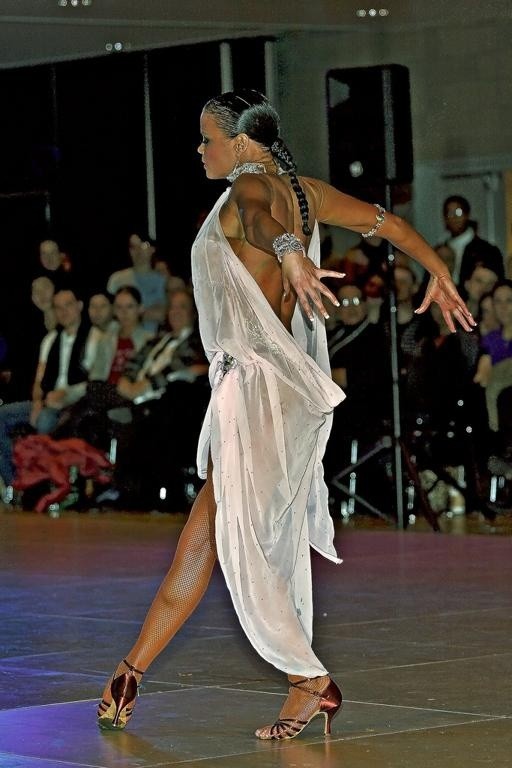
<box><xmin>330</xmin><ymin>185</ymin><xmax>496</xmax><ymax>531</ymax></box>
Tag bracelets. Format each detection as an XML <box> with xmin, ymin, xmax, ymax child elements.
<box><xmin>272</xmin><ymin>232</ymin><xmax>304</xmax><ymax>263</ymax></box>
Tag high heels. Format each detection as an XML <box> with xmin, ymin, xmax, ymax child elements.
<box><xmin>97</xmin><ymin>658</ymin><xmax>144</xmax><ymax>730</ymax></box>
<box><xmin>255</xmin><ymin>675</ymin><xmax>342</xmax><ymax>742</ymax></box>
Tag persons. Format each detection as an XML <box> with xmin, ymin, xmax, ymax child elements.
<box><xmin>96</xmin><ymin>88</ymin><xmax>477</xmax><ymax>740</ymax></box>
<box><xmin>321</xmin><ymin>236</ymin><xmax>511</xmax><ymax>524</ymax></box>
<box><xmin>2</xmin><ymin>236</ymin><xmax>212</xmax><ymax>513</ymax></box>
<box><xmin>420</xmin><ymin>193</ymin><xmax>508</xmax><ymax>307</ymax></box>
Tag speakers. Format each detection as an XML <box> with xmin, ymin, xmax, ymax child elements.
<box><xmin>326</xmin><ymin>64</ymin><xmax>414</xmax><ymax>185</ymax></box>
